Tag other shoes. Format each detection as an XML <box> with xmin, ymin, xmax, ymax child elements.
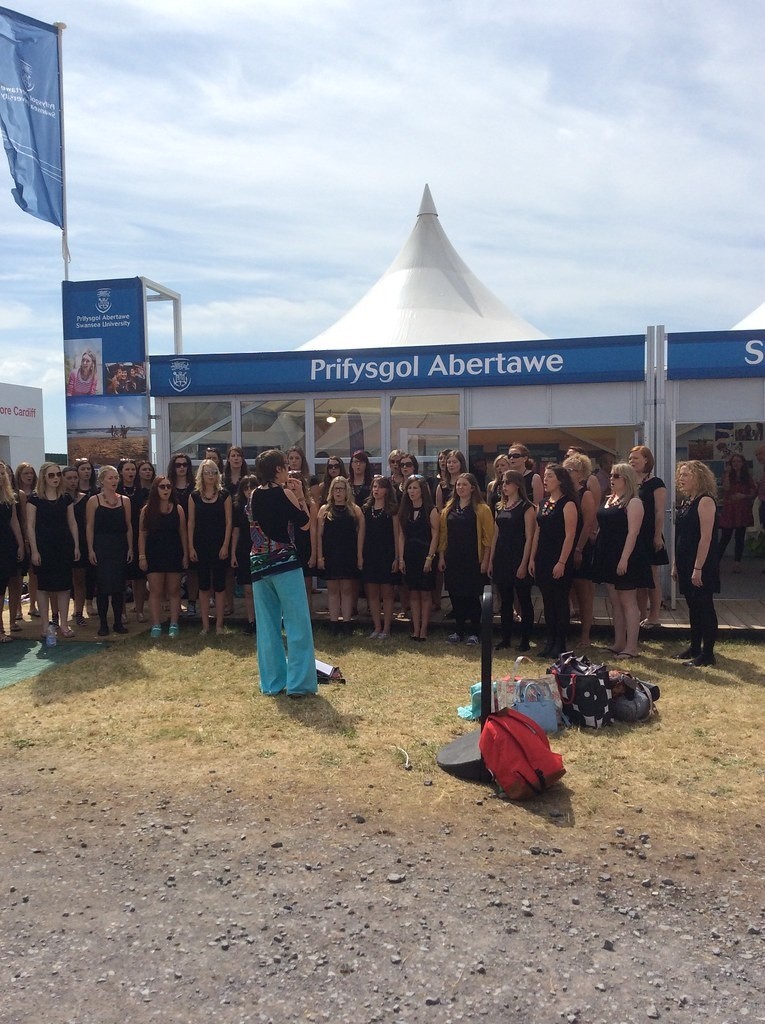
<box><xmin>732</xmin><ymin>560</ymin><xmax>742</xmax><ymax>573</ymax></box>
<box><xmin>291</xmin><ymin>691</ymin><xmax>316</xmax><ymax>699</ymax></box>
<box><xmin>0</xmin><ymin>599</ymin><xmax>719</xmax><ymax>667</ymax></box>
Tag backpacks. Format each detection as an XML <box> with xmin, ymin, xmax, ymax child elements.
<box><xmin>483</xmin><ymin>707</ymin><xmax>565</xmax><ymax>800</ymax></box>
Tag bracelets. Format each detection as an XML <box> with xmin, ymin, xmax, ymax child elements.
<box><xmin>139</xmin><ymin>554</ymin><xmax>145</xmax><ymax>560</ymax></box>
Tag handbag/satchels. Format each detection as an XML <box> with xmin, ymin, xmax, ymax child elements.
<box><xmin>547</xmin><ymin>651</ymin><xmax>614</xmax><ymax>729</ymax></box>
<box><xmin>498</xmin><ymin>656</ymin><xmax>563</xmax><ymax>725</ymax></box>
<box><xmin>608</xmin><ymin>670</ymin><xmax>661</xmax><ymax>722</ymax></box>
<box><xmin>511</xmin><ymin>679</ymin><xmax>559</xmax><ymax>732</ymax></box>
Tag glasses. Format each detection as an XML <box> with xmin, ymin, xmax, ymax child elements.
<box><xmin>507</xmin><ymin>453</ymin><xmax>523</xmax><ymax>459</ymax></box>
<box><xmin>608</xmin><ymin>474</ymin><xmax>623</xmax><ymax>479</ymax></box>
<box><xmin>333</xmin><ymin>487</ymin><xmax>347</xmax><ymax>492</ymax></box>
<box><xmin>328</xmin><ymin>464</ymin><xmax>340</xmax><ymax>469</ymax></box>
<box><xmin>408</xmin><ymin>474</ymin><xmax>424</xmax><ymax>479</ymax></box>
<box><xmin>242</xmin><ymin>485</ymin><xmax>257</xmax><ymax>490</ymax></box>
<box><xmin>400</xmin><ymin>462</ymin><xmax>415</xmax><ymax>467</ymax></box>
<box><xmin>158</xmin><ymin>484</ymin><xmax>173</xmax><ymax>490</ymax></box>
<box><xmin>121</xmin><ymin>458</ymin><xmax>135</xmax><ymax>462</ymax></box>
<box><xmin>567</xmin><ymin>468</ymin><xmax>578</xmax><ymax>473</ymax></box>
<box><xmin>45</xmin><ymin>472</ymin><xmax>62</xmax><ymax>478</ymax></box>
<box><xmin>76</xmin><ymin>457</ymin><xmax>89</xmax><ymax>462</ymax></box>
<box><xmin>203</xmin><ymin>469</ymin><xmax>217</xmax><ymax>476</ymax></box>
<box><xmin>207</xmin><ymin>448</ymin><xmax>217</xmax><ymax>451</ymax></box>
<box><xmin>564</xmin><ymin>454</ymin><xmax>570</xmax><ymax>459</ymax></box>
<box><xmin>374</xmin><ymin>474</ymin><xmax>386</xmax><ymax>479</ymax></box>
<box><xmin>391</xmin><ymin>460</ymin><xmax>400</xmax><ymax>464</ymax></box>
<box><xmin>288</xmin><ymin>470</ymin><xmax>301</xmax><ymax>474</ymax></box>
<box><xmin>175</xmin><ymin>462</ymin><xmax>188</xmax><ymax>467</ymax></box>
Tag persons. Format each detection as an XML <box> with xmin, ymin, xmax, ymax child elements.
<box><xmin>71</xmin><ymin>457</ymin><xmax>100</xmax><ymax>618</ymax></box>
<box><xmin>232</xmin><ymin>473</ymin><xmax>260</xmax><ymax>635</ymax></box>
<box><xmin>108</xmin><ymin>424</ymin><xmax>129</xmax><ymax>439</ymax></box>
<box><xmin>244</xmin><ymin>449</ymin><xmax>320</xmax><ymax>699</ymax></box>
<box><xmin>205</xmin><ymin>447</ymin><xmax>224</xmax><ymax>478</ymax></box>
<box><xmin>64</xmin><ymin>348</ymin><xmax>98</xmax><ymax>398</ymax></box>
<box><xmin>136</xmin><ymin>474</ymin><xmax>190</xmax><ymax>640</ymax></box>
<box><xmin>0</xmin><ymin>462</ymin><xmax>25</xmax><ymax>643</ymax></box>
<box><xmin>87</xmin><ymin>465</ymin><xmax>134</xmax><ymax>638</ymax></box>
<box><xmin>26</xmin><ymin>462</ymin><xmax>80</xmax><ymax>640</ymax></box>
<box><xmin>10</xmin><ymin>462</ymin><xmax>43</xmax><ymax>621</ymax></box>
<box><xmin>166</xmin><ymin>453</ymin><xmax>200</xmax><ymax>617</ymax></box>
<box><xmin>117</xmin><ymin>458</ymin><xmax>148</xmax><ymax>625</ymax></box>
<box><xmin>689</xmin><ymin>422</ymin><xmax>764</xmax><ymax>462</ymax></box>
<box><xmin>50</xmin><ymin>466</ymin><xmax>89</xmax><ymax>633</ymax></box>
<box><xmin>138</xmin><ymin>460</ymin><xmax>156</xmax><ymax>490</ymax></box>
<box><xmin>188</xmin><ymin>458</ymin><xmax>233</xmax><ymax>640</ymax></box>
<box><xmin>5</xmin><ymin>464</ymin><xmax>26</xmax><ymax>542</ymax></box>
<box><xmin>106</xmin><ymin>362</ymin><xmax>146</xmax><ymax>395</ymax></box>
<box><xmin>222</xmin><ymin>444</ymin><xmax>250</xmax><ymax>507</ymax></box>
<box><xmin>282</xmin><ymin>442</ymin><xmax>764</xmax><ymax>668</ymax></box>
